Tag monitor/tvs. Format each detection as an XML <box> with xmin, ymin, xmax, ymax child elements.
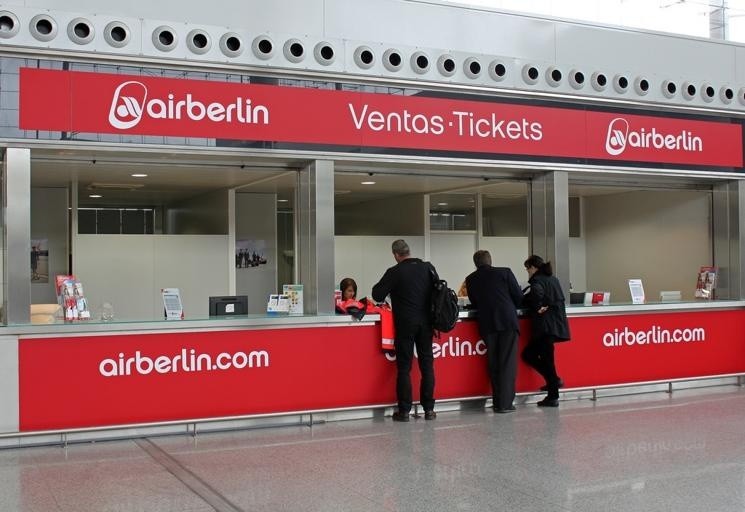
<box><xmin>208</xmin><ymin>296</ymin><xmax>248</xmax><ymax>316</ymax></box>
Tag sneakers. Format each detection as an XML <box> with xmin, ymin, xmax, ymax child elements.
<box><xmin>540</xmin><ymin>377</ymin><xmax>563</xmax><ymax>391</ymax></box>
<box><xmin>493</xmin><ymin>404</ymin><xmax>517</xmax><ymax>413</ymax></box>
<box><xmin>537</xmin><ymin>396</ymin><xmax>560</xmax><ymax>408</ymax></box>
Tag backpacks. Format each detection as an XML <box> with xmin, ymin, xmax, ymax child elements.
<box><xmin>427</xmin><ymin>262</ymin><xmax>461</xmax><ymax>334</ymax></box>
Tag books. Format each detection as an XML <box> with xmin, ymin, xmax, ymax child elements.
<box><xmin>695</xmin><ymin>265</ymin><xmax>720</xmax><ymax>298</ymax></box>
<box><xmin>54</xmin><ymin>275</ymin><xmax>90</xmax><ymax>321</ymax></box>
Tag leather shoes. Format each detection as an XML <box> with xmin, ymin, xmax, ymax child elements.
<box><xmin>425</xmin><ymin>410</ymin><xmax>437</xmax><ymax>420</ymax></box>
<box><xmin>391</xmin><ymin>409</ymin><xmax>410</xmax><ymax>422</ymax></box>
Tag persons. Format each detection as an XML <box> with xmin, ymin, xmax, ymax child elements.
<box><xmin>243</xmin><ymin>247</ymin><xmax>250</xmax><ymax>268</ymax></box>
<box><xmin>238</xmin><ymin>248</ymin><xmax>243</xmax><ymax>268</ymax></box>
<box><xmin>29</xmin><ymin>246</ymin><xmax>41</xmax><ymax>281</ymax></box>
<box><xmin>340</xmin><ymin>278</ymin><xmax>358</xmax><ymax>301</ymax></box>
<box><xmin>458</xmin><ymin>281</ymin><xmax>468</xmax><ymax>298</ymax></box>
<box><xmin>252</xmin><ymin>251</ymin><xmax>256</xmax><ymax>261</ymax></box>
<box><xmin>466</xmin><ymin>249</ymin><xmax>527</xmax><ymax>412</ymax></box>
<box><xmin>256</xmin><ymin>253</ymin><xmax>259</xmax><ymax>261</ymax></box>
<box><xmin>521</xmin><ymin>255</ymin><xmax>572</xmax><ymax>406</ymax></box>
<box><xmin>371</xmin><ymin>239</ymin><xmax>460</xmax><ymax>421</ymax></box>
